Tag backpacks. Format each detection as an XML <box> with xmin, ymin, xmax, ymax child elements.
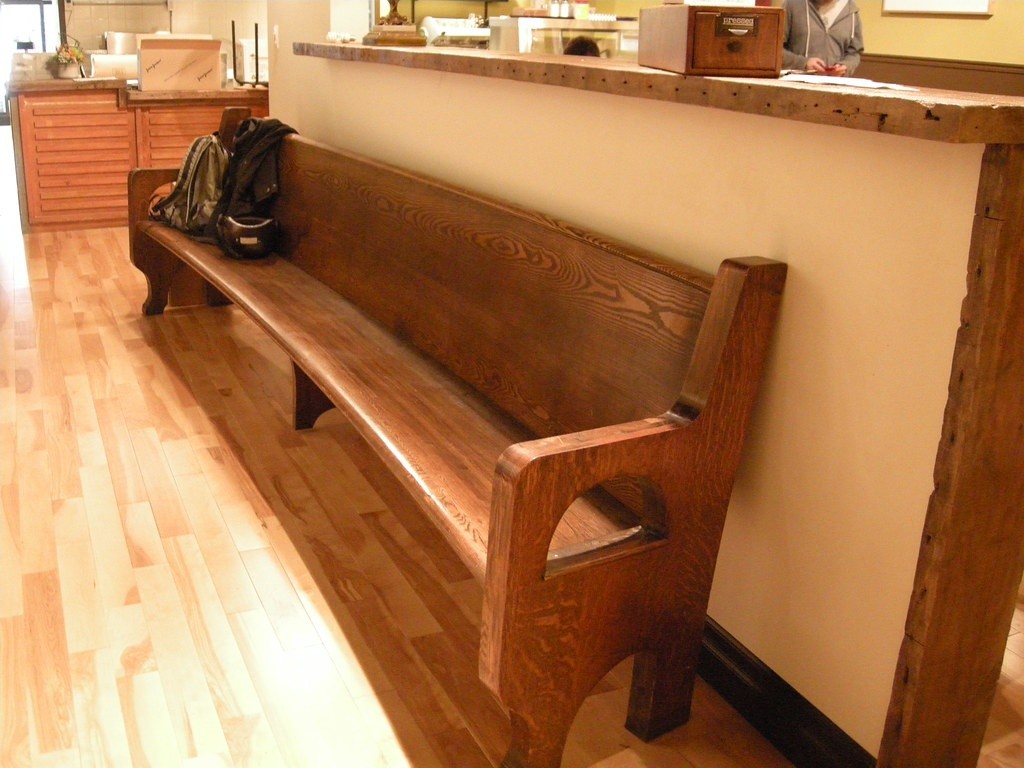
<box><xmin>148</xmin><ymin>131</ymin><xmax>232</xmax><ymax>233</ymax></box>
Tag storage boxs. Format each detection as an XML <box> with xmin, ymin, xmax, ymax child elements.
<box><xmin>637</xmin><ymin>2</ymin><xmax>784</xmax><ymax>76</ymax></box>
<box><xmin>138</xmin><ymin>35</ymin><xmax>224</xmax><ymax>92</ymax></box>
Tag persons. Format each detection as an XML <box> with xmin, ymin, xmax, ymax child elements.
<box><xmin>563</xmin><ymin>36</ymin><xmax>601</xmax><ymax>56</ymax></box>
<box><xmin>779</xmin><ymin>0</ymin><xmax>865</xmax><ymax>79</ymax></box>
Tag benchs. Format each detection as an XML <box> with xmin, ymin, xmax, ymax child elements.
<box><xmin>123</xmin><ymin>108</ymin><xmax>788</xmax><ymax>768</ymax></box>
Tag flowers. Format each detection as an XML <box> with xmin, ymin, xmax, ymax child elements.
<box><xmin>52</xmin><ymin>43</ymin><xmax>86</xmax><ymax>65</ymax></box>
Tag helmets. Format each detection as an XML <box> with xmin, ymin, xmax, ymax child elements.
<box><xmin>217</xmin><ymin>214</ymin><xmax>282</xmax><ymax>259</ymax></box>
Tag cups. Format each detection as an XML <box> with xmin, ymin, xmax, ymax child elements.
<box><xmin>573</xmin><ymin>3</ymin><xmax>590</xmax><ymax>21</ymax></box>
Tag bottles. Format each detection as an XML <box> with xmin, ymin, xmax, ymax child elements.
<box><xmin>549</xmin><ymin>0</ymin><xmax>569</xmax><ymax>18</ymax></box>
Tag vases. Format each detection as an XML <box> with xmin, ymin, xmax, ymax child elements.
<box><xmin>54</xmin><ymin>63</ymin><xmax>81</xmax><ymax>80</ymax></box>
